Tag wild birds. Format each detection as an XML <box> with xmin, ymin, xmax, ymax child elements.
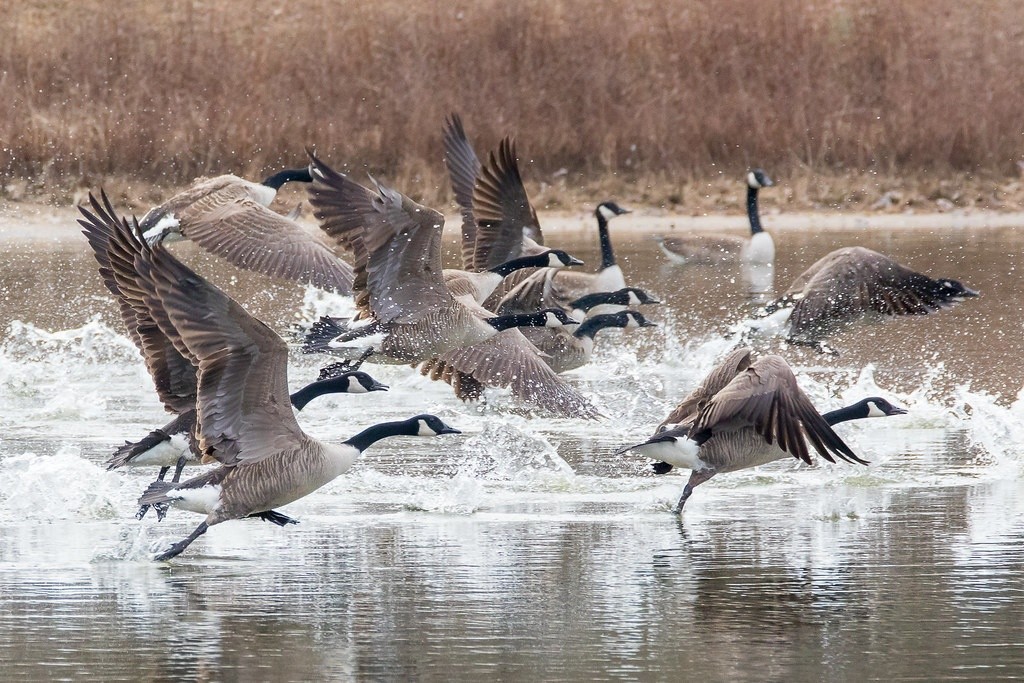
<box><xmin>659</xmin><ymin>170</ymin><xmax>978</xmax><ymax>356</ymax></box>
<box><xmin>76</xmin><ymin>113</ymin><xmax>657</xmax><ymax>564</ymax></box>
<box><xmin>612</xmin><ymin>349</ymin><xmax>907</xmax><ymax>513</ymax></box>
